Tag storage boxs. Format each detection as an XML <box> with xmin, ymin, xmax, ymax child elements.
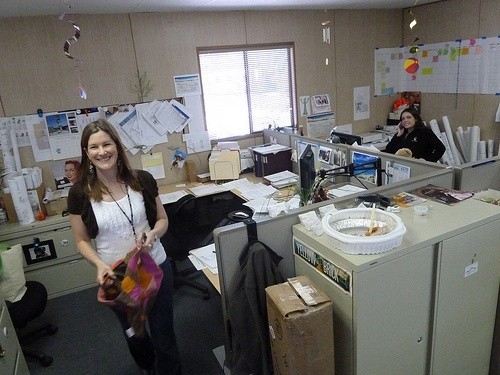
<box><xmin>264</xmin><ymin>275</ymin><xmax>334</xmax><ymax>375</ymax></box>
<box><xmin>186</xmin><ymin>160</ymin><xmax>197</xmax><ymax>184</ymax></box>
<box><xmin>248</xmin><ymin>143</ymin><xmax>293</xmax><ymax>177</ymax></box>
<box><xmin>1</xmin><ymin>181</ymin><xmax>69</xmax><ymax>223</ymax></box>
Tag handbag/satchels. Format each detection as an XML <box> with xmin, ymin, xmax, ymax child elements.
<box><xmin>97</xmin><ymin>245</ymin><xmax>164</xmax><ymax>337</ymax></box>
<box><xmin>0</xmin><ymin>243</ymin><xmax>27</xmax><ymax>303</ymax></box>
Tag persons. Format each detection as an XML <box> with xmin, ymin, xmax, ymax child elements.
<box><xmin>68</xmin><ymin>120</ymin><xmax>182</xmax><ymax>375</ymax></box>
<box><xmin>384</xmin><ymin>107</ymin><xmax>446</xmax><ymax>163</ymax></box>
<box><xmin>65</xmin><ymin>160</ymin><xmax>81</xmax><ymax>185</ymax></box>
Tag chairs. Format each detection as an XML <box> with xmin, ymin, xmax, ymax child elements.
<box><xmin>6</xmin><ymin>280</ymin><xmax>59</xmax><ymax>367</ymax></box>
<box><xmin>159</xmin><ymin>194</ymin><xmax>212</xmax><ymax>301</ymax></box>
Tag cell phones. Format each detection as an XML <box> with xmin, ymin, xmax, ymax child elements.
<box><xmin>399</xmin><ymin>123</ymin><xmax>403</xmax><ymax>129</ymax></box>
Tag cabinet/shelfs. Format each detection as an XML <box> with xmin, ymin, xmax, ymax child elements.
<box><xmin>292</xmin><ymin>194</ymin><xmax>500</xmax><ymax>375</ymax></box>
<box><xmin>0</xmin><ymin>213</ymin><xmax>104</xmax><ymax>300</ymax></box>
<box><xmin>0</xmin><ymin>293</ymin><xmax>31</xmax><ymax>375</ymax></box>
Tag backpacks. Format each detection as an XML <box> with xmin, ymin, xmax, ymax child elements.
<box><xmin>223</xmin><ymin>219</ymin><xmax>286</xmax><ymax>375</ymax></box>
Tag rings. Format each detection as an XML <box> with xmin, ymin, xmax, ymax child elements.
<box><xmin>149</xmin><ymin>244</ymin><xmax>152</xmax><ymax>247</ymax></box>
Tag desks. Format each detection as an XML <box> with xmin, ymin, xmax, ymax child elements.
<box><xmin>158</xmin><ymin>170</ymin><xmax>301</xmax><ymax>295</ymax></box>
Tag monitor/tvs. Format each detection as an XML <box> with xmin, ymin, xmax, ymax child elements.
<box><xmin>297</xmin><ymin>144</ymin><xmax>317</xmax><ymax>206</ymax></box>
<box><xmin>349</xmin><ymin>149</ymin><xmax>382</xmax><ymax>189</ymax></box>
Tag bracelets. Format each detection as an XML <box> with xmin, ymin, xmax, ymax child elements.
<box><xmin>151</xmin><ymin>230</ymin><xmax>157</xmax><ymax>242</ymax></box>
